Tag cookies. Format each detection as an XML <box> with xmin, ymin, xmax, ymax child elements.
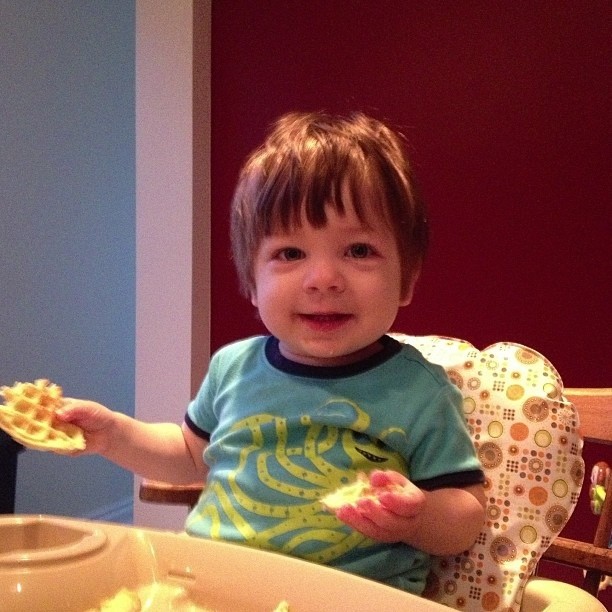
<box><xmin>320</xmin><ymin>479</ymin><xmax>407</xmax><ymax>513</ymax></box>
<box><xmin>0</xmin><ymin>375</ymin><xmax>90</xmax><ymax>456</ymax></box>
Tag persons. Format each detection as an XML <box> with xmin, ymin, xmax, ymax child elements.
<box><xmin>53</xmin><ymin>112</ymin><xmax>487</xmax><ymax>594</ymax></box>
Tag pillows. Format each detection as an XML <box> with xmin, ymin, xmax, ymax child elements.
<box><xmin>382</xmin><ymin>333</ymin><xmax>586</xmax><ymax>611</ymax></box>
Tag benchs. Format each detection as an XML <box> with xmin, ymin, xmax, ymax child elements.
<box><xmin>139</xmin><ymin>389</ymin><xmax>612</xmax><ymax>611</ymax></box>
